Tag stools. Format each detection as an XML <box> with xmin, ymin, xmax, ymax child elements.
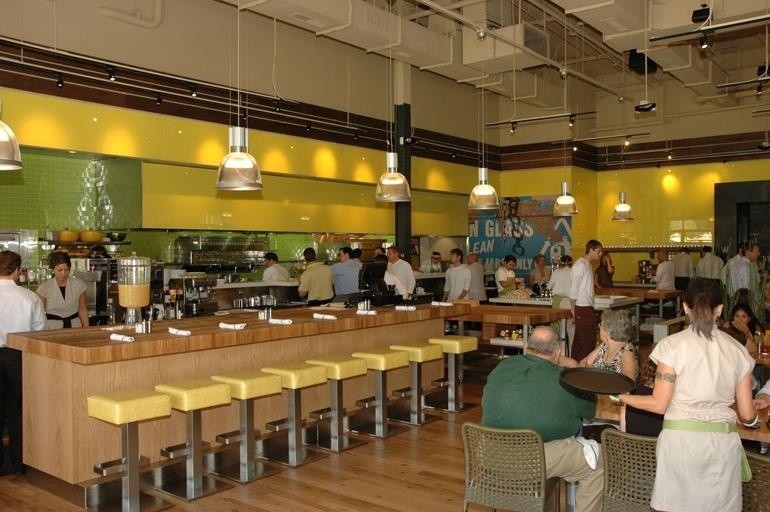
<box><xmin>142</xmin><ymin>378</ymin><xmax>232</xmax><ymax>503</ymax></box>
<box><xmin>350</xmin><ymin>350</ymin><xmax>411</xmax><ymax>438</ymax></box>
<box><xmin>387</xmin><ymin>340</ymin><xmax>444</xmax><ymax>427</ymax></box>
<box><xmin>428</xmin><ymin>335</ymin><xmax>478</xmax><ymax>414</ymax></box>
<box><xmin>82</xmin><ymin>389</ymin><xmax>174</xmax><ymax>508</ymax></box>
<box><xmin>303</xmin><ymin>355</ymin><xmax>368</xmax><ymax>453</ymax></box>
<box><xmin>259</xmin><ymin>362</ymin><xmax>329</xmax><ymax>467</ymax></box>
<box><xmin>443</xmin><ymin>286</ymin><xmax>770</xmax><ymax>510</ymax></box>
<box><xmin>203</xmin><ymin>372</ymin><xmax>285</xmax><ymax>484</ymax></box>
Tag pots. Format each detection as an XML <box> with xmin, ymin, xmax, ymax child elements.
<box><xmin>58</xmin><ymin>228</ymin><xmax>80</xmax><ymax>242</ymax></box>
<box><xmin>79</xmin><ymin>228</ymin><xmax>103</xmax><ymax>242</ymax></box>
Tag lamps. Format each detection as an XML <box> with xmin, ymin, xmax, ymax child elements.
<box><xmin>212</xmin><ymin>0</ymin><xmax>268</xmax><ymax>194</ymax></box>
<box><xmin>550</xmin><ymin>68</ymin><xmax>579</xmax><ymax>218</ymax></box>
<box><xmin>464</xmin><ymin>25</ymin><xmax>504</xmax><ymax>212</ymax></box>
<box><xmin>372</xmin><ymin>1</ymin><xmax>416</xmax><ymax>203</ymax></box>
<box><xmin>608</xmin><ymin>95</ymin><xmax>635</xmax><ymax>222</ymax></box>
<box><xmin>0</xmin><ymin>119</ymin><xmax>26</xmax><ymax>173</ymax></box>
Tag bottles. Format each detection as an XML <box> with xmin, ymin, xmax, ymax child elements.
<box><xmin>142</xmin><ymin>318</ymin><xmax>153</xmax><ymax>335</ymax></box>
<box><xmin>505</xmin><ymin>326</ymin><xmax>533</xmax><ymax>341</ymax></box>
<box><xmin>364</xmin><ymin>299</ymin><xmax>371</xmax><ymax>310</ymax></box>
<box><xmin>264</xmin><ymin>307</ymin><xmax>273</xmax><ymax>320</ymax></box>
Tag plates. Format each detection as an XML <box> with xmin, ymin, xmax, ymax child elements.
<box><xmin>560</xmin><ymin>365</ymin><xmax>638</xmax><ymax>395</ymax></box>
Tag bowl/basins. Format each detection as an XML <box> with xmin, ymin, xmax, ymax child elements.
<box><xmin>106</xmin><ymin>231</ymin><xmax>127</xmax><ymax>241</ymax></box>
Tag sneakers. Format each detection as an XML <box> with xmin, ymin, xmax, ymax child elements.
<box><xmin>14</xmin><ymin>463</ymin><xmax>26</xmax><ymax>476</ymax></box>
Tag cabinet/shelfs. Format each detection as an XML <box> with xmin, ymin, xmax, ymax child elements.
<box><xmin>7</xmin><ymin>303</ymin><xmax>473</xmax><ymax>484</ymax></box>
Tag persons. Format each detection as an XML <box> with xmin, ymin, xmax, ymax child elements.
<box><xmin>671</xmin><ymin>248</ymin><xmax>695</xmax><ymax>290</ymax></box>
<box><xmin>495</xmin><ymin>255</ymin><xmax>525</xmax><ymax>296</ymax></box>
<box><xmin>719</xmin><ymin>242</ymin><xmax>766</xmax><ymax>331</ymax></box>
<box><xmin>579</xmin><ymin>309</ymin><xmax>640</xmax><ymax>394</ymax></box>
<box><xmin>352</xmin><ymin>246</ymin><xmax>416</xmax><ymax>300</ymax></box>
<box><xmin>35</xmin><ymin>251</ymin><xmax>90</xmax><ymax>329</ymax></box>
<box><xmin>569</xmin><ymin>240</ymin><xmax>603</xmax><ymax>362</ymax></box>
<box><xmin>419</xmin><ymin>251</ymin><xmax>442</xmax><ymax>273</ymax></box>
<box><xmin>527</xmin><ymin>255</ymin><xmax>552</xmax><ymax>289</ymax></box>
<box><xmin>331</xmin><ymin>246</ymin><xmax>363</xmax><ymax>302</ymax></box>
<box><xmin>442</xmin><ymin>248</ymin><xmax>472</xmax><ymax>303</ymax></box>
<box><xmin>300</xmin><ymin>247</ymin><xmax>334</xmax><ymax>307</ymax></box>
<box><xmin>466</xmin><ymin>252</ymin><xmax>488</xmax><ymax>304</ymax></box>
<box><xmin>719</xmin><ymin>302</ymin><xmax>764</xmax><ymax>355</ymax></box>
<box><xmin>610</xmin><ymin>277</ymin><xmax>759</xmax><ymax>511</ymax></box>
<box><xmin>650</xmin><ymin>249</ymin><xmax>675</xmax><ymax>291</ymax></box>
<box><xmin>696</xmin><ymin>246</ymin><xmax>723</xmax><ymax>281</ymax></box>
<box><xmin>478</xmin><ymin>326</ymin><xmax>605</xmax><ymax>511</ymax></box>
<box><xmin>594</xmin><ymin>254</ymin><xmax>615</xmax><ymax>289</ymax></box>
<box><xmin>546</xmin><ymin>255</ymin><xmax>576</xmax><ymax>358</ymax></box>
<box><xmin>0</xmin><ymin>250</ymin><xmax>48</xmax><ymax>475</ymax></box>
<box><xmin>263</xmin><ymin>253</ymin><xmax>291</xmax><ymax>282</ymax></box>
